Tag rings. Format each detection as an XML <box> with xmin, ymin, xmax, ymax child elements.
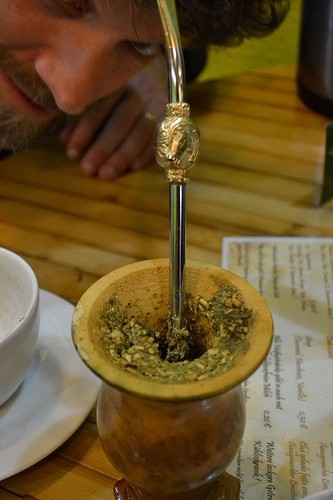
<box><xmin>139</xmin><ymin>110</ymin><xmax>159</xmax><ymax>124</ymax></box>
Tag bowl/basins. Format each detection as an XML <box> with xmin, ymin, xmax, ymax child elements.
<box><xmin>0</xmin><ymin>242</ymin><xmax>44</xmax><ymax>412</ymax></box>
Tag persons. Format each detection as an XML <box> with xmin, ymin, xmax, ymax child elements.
<box><xmin>0</xmin><ymin>0</ymin><xmax>292</xmax><ymax>177</ymax></box>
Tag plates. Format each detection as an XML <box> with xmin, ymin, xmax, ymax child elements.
<box><xmin>0</xmin><ymin>284</ymin><xmax>105</xmax><ymax>486</ymax></box>
